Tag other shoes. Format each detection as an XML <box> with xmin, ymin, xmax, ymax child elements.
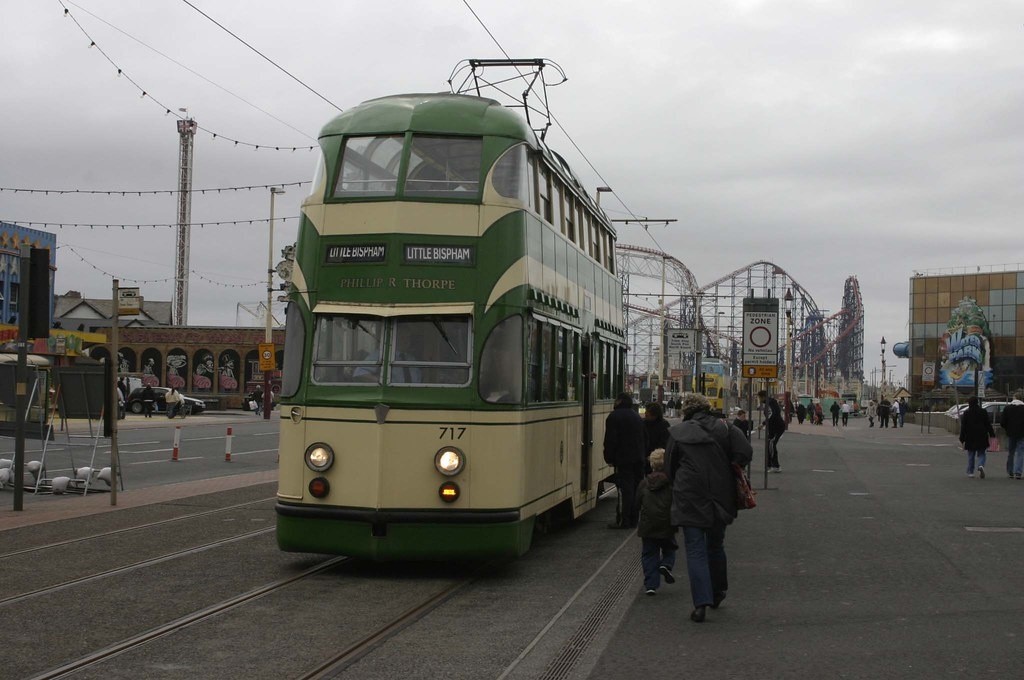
<box><xmin>658</xmin><ymin>566</ymin><xmax>676</xmax><ymax>585</ymax></box>
<box><xmin>978</xmin><ymin>466</ymin><xmax>986</xmax><ymax>479</ymax></box>
<box><xmin>608</xmin><ymin>521</ymin><xmax>638</xmax><ymax>529</ymax></box>
<box><xmin>646</xmin><ymin>587</ymin><xmax>656</xmax><ymax>596</ymax></box>
<box><xmin>767</xmin><ymin>466</ymin><xmax>782</xmax><ymax>473</ymax></box>
<box><xmin>710</xmin><ymin>592</ymin><xmax>727</xmax><ymax>609</ymax></box>
<box><xmin>691</xmin><ymin>607</ymin><xmax>707</xmax><ymax>622</ymax></box>
<box><xmin>968</xmin><ymin>474</ymin><xmax>975</xmax><ymax>477</ymax></box>
<box><xmin>1008</xmin><ymin>473</ymin><xmax>1022</xmax><ymax>479</ymax></box>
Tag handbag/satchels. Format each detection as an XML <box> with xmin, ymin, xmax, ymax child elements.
<box><xmin>733</xmin><ymin>464</ymin><xmax>756</xmax><ymax>511</ymax></box>
<box><xmin>987</xmin><ymin>438</ymin><xmax>1000</xmax><ymax>452</ymax></box>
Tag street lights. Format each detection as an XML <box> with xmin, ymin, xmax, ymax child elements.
<box><xmin>264</xmin><ymin>187</ymin><xmax>286</xmax><ymax>419</ymax></box>
<box><xmin>657</xmin><ymin>255</ymin><xmax>674</xmax><ymax>404</ymax></box>
<box><xmin>717</xmin><ymin>312</ymin><xmax>725</xmax><ymax>336</ymax></box>
<box><xmin>726</xmin><ymin>325</ymin><xmax>736</xmax><ymax>357</ymax></box>
<box><xmin>880</xmin><ymin>337</ymin><xmax>886</xmax><ymax>401</ymax></box>
<box><xmin>596</xmin><ymin>186</ymin><xmax>612</xmax><ymax>206</ymax></box>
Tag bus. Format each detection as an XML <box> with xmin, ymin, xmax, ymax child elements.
<box><xmin>691</xmin><ymin>360</ymin><xmax>731</xmax><ymax>418</ymax></box>
<box><xmin>274</xmin><ymin>91</ymin><xmax>632</xmax><ymax>559</ymax></box>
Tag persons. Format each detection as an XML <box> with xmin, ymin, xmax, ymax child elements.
<box><xmin>865</xmin><ymin>396</ymin><xmax>909</xmax><ymax>429</ymax></box>
<box><xmin>166</xmin><ymin>386</ymin><xmax>180</xmax><ymax>413</ymax></box>
<box><xmin>582</xmin><ymin>371</ymin><xmax>597</xmax><ymax>382</ymax></box>
<box><xmin>841</xmin><ymin>401</ymin><xmax>849</xmax><ymax>427</ymax></box>
<box><xmin>853</xmin><ymin>401</ymin><xmax>859</xmax><ymax>417</ymax></box>
<box><xmin>829</xmin><ymin>401</ymin><xmax>841</xmax><ymax>427</ymax></box>
<box><xmin>252</xmin><ymin>385</ymin><xmax>274</xmax><ymax>417</ymax></box>
<box><xmin>958</xmin><ymin>397</ymin><xmax>995</xmax><ymax>479</ymax></box>
<box><xmin>638</xmin><ymin>449</ymin><xmax>678</xmax><ymax>595</ymax></box>
<box><xmin>354</xmin><ymin>326</ymin><xmax>421</xmax><ymax>385</ymax></box>
<box><xmin>1001</xmin><ymin>390</ymin><xmax>1024</xmax><ymax>479</ymax></box>
<box><xmin>644</xmin><ymin>403</ymin><xmax>671</xmax><ymax>468</ymax></box>
<box><xmin>667</xmin><ymin>397</ymin><xmax>682</xmax><ymax>418</ymax></box>
<box><xmin>797</xmin><ymin>404</ymin><xmax>805</xmax><ymax>424</ymax></box>
<box><xmin>664</xmin><ymin>393</ymin><xmax>754</xmax><ymax>625</ymax></box>
<box><xmin>117</xmin><ymin>376</ymin><xmax>127</xmax><ymax>419</ymax></box>
<box><xmin>808</xmin><ymin>399</ymin><xmax>824</xmax><ymax>425</ymax></box>
<box><xmin>604</xmin><ymin>394</ymin><xmax>643</xmax><ymax>528</ymax></box>
<box><xmin>141</xmin><ymin>383</ymin><xmax>157</xmax><ymax>418</ymax></box>
<box><xmin>732</xmin><ymin>410</ymin><xmax>748</xmax><ymax>439</ymax></box>
<box><xmin>440</xmin><ymin>331</ymin><xmax>511</xmax><ymax>392</ymax></box>
<box><xmin>756</xmin><ymin>390</ymin><xmax>785</xmax><ymax>473</ymax></box>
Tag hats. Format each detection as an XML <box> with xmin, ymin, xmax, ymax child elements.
<box><xmin>650</xmin><ymin>448</ymin><xmax>665</xmax><ymax>468</ymax></box>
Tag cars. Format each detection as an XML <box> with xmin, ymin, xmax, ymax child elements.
<box><xmin>948</xmin><ymin>400</ymin><xmax>1012</xmax><ymax>424</ymax></box>
<box><xmin>126</xmin><ymin>387</ymin><xmax>206</xmax><ymax>417</ymax></box>
